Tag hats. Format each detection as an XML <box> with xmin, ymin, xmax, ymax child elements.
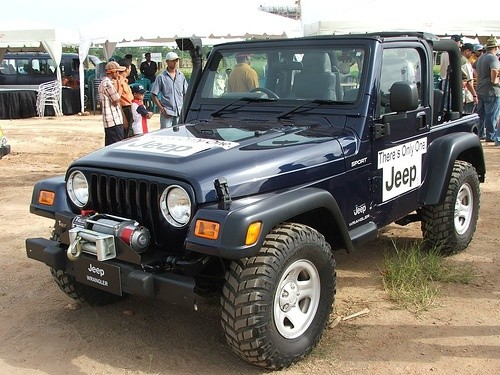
<box><xmin>125</xmin><ymin>55</ymin><xmax>132</xmax><ymax>59</ymax></box>
<box><xmin>452</xmin><ymin>35</ymin><xmax>463</xmax><ymax>41</ymax></box>
<box><xmin>462</xmin><ymin>43</ymin><xmax>476</xmax><ymax>54</ymax></box>
<box><xmin>133</xmin><ymin>85</ymin><xmax>146</xmax><ymax>94</ymax></box>
<box><xmin>105</xmin><ymin>61</ymin><xmax>126</xmax><ymax>73</ymax></box>
<box><xmin>165</xmin><ymin>52</ymin><xmax>181</xmax><ymax>61</ymax></box>
<box><xmin>473</xmin><ymin>45</ymin><xmax>484</xmax><ymax>51</ymax></box>
<box><xmin>485</xmin><ymin>38</ymin><xmax>500</xmax><ymax>47</ymax></box>
<box><xmin>235</xmin><ymin>53</ymin><xmax>250</xmax><ymax>57</ymax></box>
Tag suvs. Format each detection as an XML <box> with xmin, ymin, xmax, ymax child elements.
<box><xmin>24</xmin><ymin>31</ymin><xmax>487</xmax><ymax>372</ymax></box>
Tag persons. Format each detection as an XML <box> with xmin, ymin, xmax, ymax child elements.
<box><xmin>140</xmin><ymin>52</ymin><xmax>158</xmax><ymax>83</ymax></box>
<box><xmin>130</xmin><ymin>86</ymin><xmax>152</xmax><ymax>135</ymax></box>
<box><xmin>229</xmin><ymin>54</ymin><xmax>261</xmax><ymax>93</ymax></box>
<box><xmin>225</xmin><ymin>69</ymin><xmax>232</xmax><ymax>91</ymax></box>
<box><xmin>401</xmin><ymin>53</ymin><xmax>419</xmax><ymax>83</ymax></box>
<box><xmin>123</xmin><ymin>53</ymin><xmax>138</xmax><ymax>85</ymax></box>
<box><xmin>149</xmin><ymin>52</ymin><xmax>189</xmax><ymax>129</ymax></box>
<box><xmin>439</xmin><ymin>35</ymin><xmax>500</xmax><ymax>145</ymax></box>
<box><xmin>98</xmin><ymin>61</ymin><xmax>125</xmax><ymax>146</ymax></box>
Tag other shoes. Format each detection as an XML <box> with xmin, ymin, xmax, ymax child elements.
<box><xmin>480</xmin><ymin>136</ymin><xmax>486</xmax><ymax>139</ymax></box>
<box><xmin>486</xmin><ymin>140</ymin><xmax>496</xmax><ymax>142</ymax></box>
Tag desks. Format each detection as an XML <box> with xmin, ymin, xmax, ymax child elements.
<box><xmin>340</xmin><ymin>83</ymin><xmax>357</xmax><ymax>86</ymax></box>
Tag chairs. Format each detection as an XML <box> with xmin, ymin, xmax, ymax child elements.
<box><xmin>289</xmin><ymin>53</ymin><xmax>336</xmax><ymax>101</ymax></box>
<box><xmin>343</xmin><ymin>76</ymin><xmax>357</xmax><ymax>94</ymax></box>
<box><xmin>36</xmin><ymin>81</ymin><xmax>62</xmax><ymax>118</ymax></box>
<box><xmin>3</xmin><ymin>63</ymin><xmax>52</xmax><ymax>74</ymax></box>
<box><xmin>433</xmin><ymin>89</ymin><xmax>446</xmax><ymax>125</ymax></box>
<box><xmin>92</xmin><ymin>62</ymin><xmax>160</xmax><ymax>115</ymax></box>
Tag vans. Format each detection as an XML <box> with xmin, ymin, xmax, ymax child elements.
<box><xmin>0</xmin><ymin>51</ymin><xmax>102</xmax><ymax>85</ymax></box>
